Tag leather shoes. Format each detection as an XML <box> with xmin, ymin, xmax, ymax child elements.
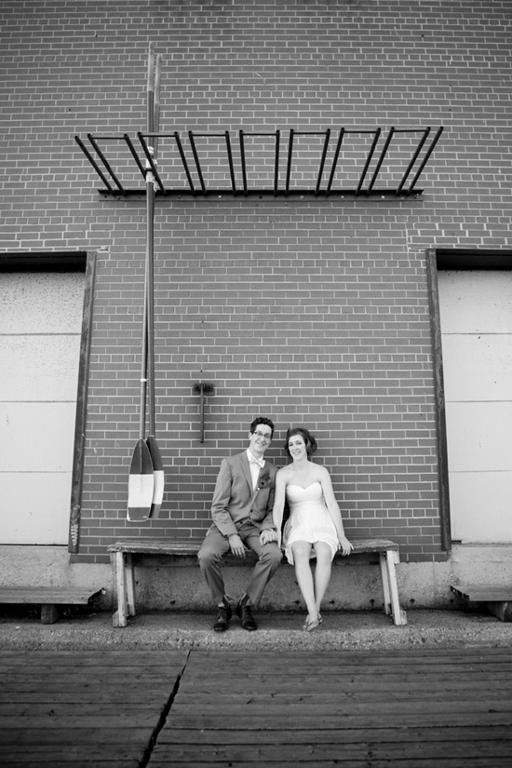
<box><xmin>235</xmin><ymin>603</ymin><xmax>258</xmax><ymax>631</ymax></box>
<box><xmin>213</xmin><ymin>607</ymin><xmax>233</xmax><ymax>632</ymax></box>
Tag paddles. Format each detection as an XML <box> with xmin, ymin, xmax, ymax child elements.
<box><xmin>125</xmin><ymin>51</ymin><xmax>165</xmax><ymax>524</ymax></box>
<box><xmin>146</xmin><ymin>39</ymin><xmax>165</xmax><ymax>517</ymax></box>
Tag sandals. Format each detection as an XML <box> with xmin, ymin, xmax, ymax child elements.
<box><xmin>303</xmin><ymin>612</ymin><xmax>324</xmax><ymax>631</ymax></box>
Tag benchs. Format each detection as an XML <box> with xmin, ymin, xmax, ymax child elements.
<box><xmin>105</xmin><ymin>539</ymin><xmax>406</xmax><ymax>625</ymax></box>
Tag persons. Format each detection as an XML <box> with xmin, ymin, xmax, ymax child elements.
<box><xmin>195</xmin><ymin>417</ymin><xmax>284</xmax><ymax>634</ymax></box>
<box><xmin>272</xmin><ymin>426</ymin><xmax>355</xmax><ymax>634</ymax></box>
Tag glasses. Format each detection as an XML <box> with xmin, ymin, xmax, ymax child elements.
<box><xmin>252</xmin><ymin>431</ymin><xmax>271</xmax><ymax>438</ymax></box>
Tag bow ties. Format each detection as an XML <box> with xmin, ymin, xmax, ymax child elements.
<box><xmin>249</xmin><ymin>457</ymin><xmax>266</xmax><ymax>468</ymax></box>
